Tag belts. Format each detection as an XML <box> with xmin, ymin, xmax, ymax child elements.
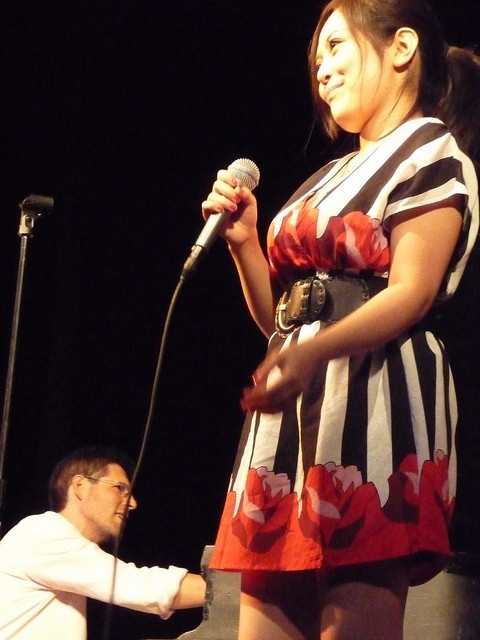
<box><xmin>266</xmin><ymin>270</ymin><xmax>389</xmax><ymax>332</ymax></box>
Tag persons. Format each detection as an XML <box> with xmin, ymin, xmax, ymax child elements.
<box><xmin>197</xmin><ymin>0</ymin><xmax>480</xmax><ymax>640</ymax></box>
<box><xmin>0</xmin><ymin>443</ymin><xmax>209</xmax><ymax>639</ymax></box>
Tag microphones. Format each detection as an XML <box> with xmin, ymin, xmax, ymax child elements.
<box><xmin>181</xmin><ymin>159</ymin><xmax>262</xmax><ymax>274</ymax></box>
